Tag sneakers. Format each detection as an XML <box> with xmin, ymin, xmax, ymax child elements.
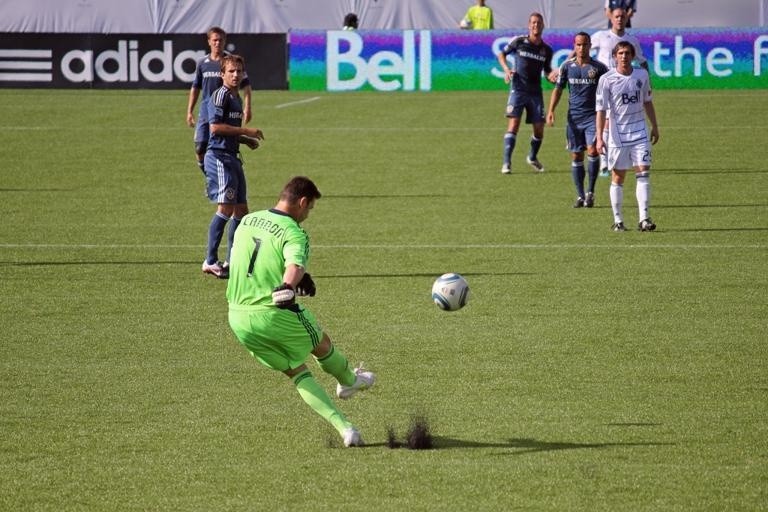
<box><xmin>613</xmin><ymin>222</ymin><xmax>628</xmax><ymax>233</ymax></box>
<box><xmin>600</xmin><ymin>167</ymin><xmax>611</xmax><ymax>178</ymax></box>
<box><xmin>526</xmin><ymin>154</ymin><xmax>545</xmax><ymax>172</ymax></box>
<box><xmin>636</xmin><ymin>219</ymin><xmax>657</xmax><ymax>232</ymax></box>
<box><xmin>501</xmin><ymin>162</ymin><xmax>512</xmax><ymax>174</ymax></box>
<box><xmin>571</xmin><ymin>196</ymin><xmax>586</xmax><ymax>209</ymax></box>
<box><xmin>222</xmin><ymin>261</ymin><xmax>231</xmax><ymax>271</ymax></box>
<box><xmin>583</xmin><ymin>192</ymin><xmax>594</xmax><ymax>207</ymax></box>
<box><xmin>336</xmin><ymin>370</ymin><xmax>376</xmax><ymax>399</ymax></box>
<box><xmin>202</xmin><ymin>258</ymin><xmax>230</xmax><ymax>279</ymax></box>
<box><xmin>342</xmin><ymin>426</ymin><xmax>365</xmax><ymax>447</ymax></box>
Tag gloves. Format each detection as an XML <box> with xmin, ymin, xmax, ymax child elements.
<box><xmin>297</xmin><ymin>272</ymin><xmax>318</xmax><ymax>298</ymax></box>
<box><xmin>272</xmin><ymin>283</ymin><xmax>296</xmax><ymax>308</ymax></box>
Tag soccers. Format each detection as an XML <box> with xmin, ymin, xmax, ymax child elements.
<box><xmin>431</xmin><ymin>272</ymin><xmax>470</xmax><ymax>312</ymax></box>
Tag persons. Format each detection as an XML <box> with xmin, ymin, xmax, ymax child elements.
<box><xmin>200</xmin><ymin>54</ymin><xmax>265</xmax><ymax>279</ymax></box>
<box><xmin>459</xmin><ymin>0</ymin><xmax>494</xmax><ymax>30</ymax></box>
<box><xmin>342</xmin><ymin>13</ymin><xmax>359</xmax><ymax>31</ymax></box>
<box><xmin>545</xmin><ymin>31</ymin><xmax>609</xmax><ymax>208</ymax></box>
<box><xmin>186</xmin><ymin>26</ymin><xmax>253</xmax><ymax>176</ymax></box>
<box><xmin>497</xmin><ymin>12</ymin><xmax>560</xmax><ymax>174</ymax></box>
<box><xmin>225</xmin><ymin>174</ymin><xmax>374</xmax><ymax>447</ymax></box>
<box><xmin>595</xmin><ymin>40</ymin><xmax>659</xmax><ymax>231</ymax></box>
<box><xmin>567</xmin><ymin>7</ymin><xmax>649</xmax><ymax>176</ymax></box>
<box><xmin>604</xmin><ymin>0</ymin><xmax>638</xmax><ymax>28</ymax></box>
